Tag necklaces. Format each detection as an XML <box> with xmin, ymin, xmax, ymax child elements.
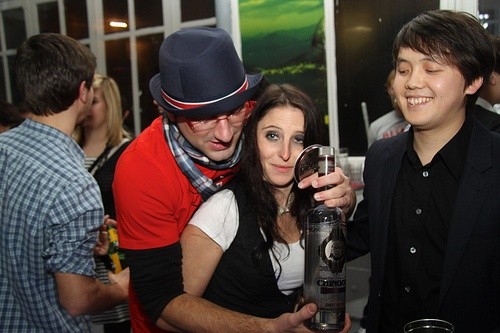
<box><xmin>277</xmin><ymin>192</ymin><xmax>295</xmax><ymax>216</ymax></box>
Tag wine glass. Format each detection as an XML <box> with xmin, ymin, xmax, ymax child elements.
<box><xmin>336</xmin><ymin>148</ymin><xmax>348</xmax><ymax>176</ymax></box>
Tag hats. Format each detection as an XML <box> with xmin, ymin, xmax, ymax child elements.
<box><xmin>149</xmin><ymin>25</ymin><xmax>263</xmax><ymax>119</ymax></box>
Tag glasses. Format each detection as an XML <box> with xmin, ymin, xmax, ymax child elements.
<box><xmin>184</xmin><ymin>100</ymin><xmax>250</xmax><ymax>132</ymax></box>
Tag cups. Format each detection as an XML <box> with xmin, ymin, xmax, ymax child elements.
<box><xmin>349</xmin><ymin>164</ymin><xmax>362</xmax><ymax>183</ymax></box>
<box><xmin>404</xmin><ymin>319</ymin><xmax>454</xmax><ymax>333</ymax></box>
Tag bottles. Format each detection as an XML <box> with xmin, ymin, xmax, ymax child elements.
<box><xmin>105</xmin><ymin>224</ymin><xmax>129</xmax><ymax>274</ymax></box>
<box><xmin>304</xmin><ymin>147</ymin><xmax>346</xmax><ymax>333</ymax></box>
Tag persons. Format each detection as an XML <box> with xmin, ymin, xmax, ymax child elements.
<box><xmin>0</xmin><ymin>33</ymin><xmax>129</xmax><ymax>333</ymax></box>
<box><xmin>467</xmin><ymin>34</ymin><xmax>499</xmax><ymax>132</ymax></box>
<box><xmin>336</xmin><ymin>10</ymin><xmax>500</xmax><ymax>333</ymax></box>
<box><xmin>74</xmin><ymin>74</ymin><xmax>134</xmax><ymax>333</ymax></box>
<box><xmin>368</xmin><ymin>70</ymin><xmax>410</xmax><ymax>149</ymax></box>
<box><xmin>0</xmin><ymin>102</ymin><xmax>22</xmax><ymax>134</ymax></box>
<box><xmin>112</xmin><ymin>27</ymin><xmax>357</xmax><ymax>333</ymax></box>
<box><xmin>155</xmin><ymin>84</ymin><xmax>321</xmax><ymax>332</ymax></box>
